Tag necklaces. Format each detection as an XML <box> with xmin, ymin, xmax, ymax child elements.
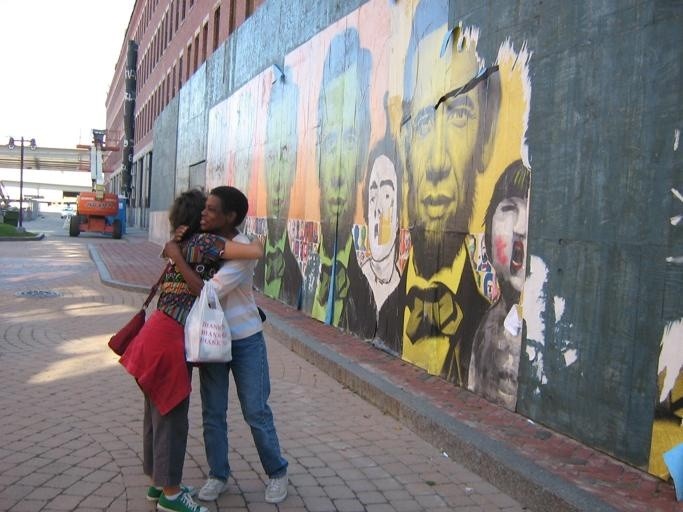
<box><xmin>364</xmin><ymin>257</ymin><xmax>397</xmax><ymax>287</ymax></box>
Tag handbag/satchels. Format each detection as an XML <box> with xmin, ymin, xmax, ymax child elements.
<box><xmin>107</xmin><ymin>307</ymin><xmax>145</xmax><ymax>356</ymax></box>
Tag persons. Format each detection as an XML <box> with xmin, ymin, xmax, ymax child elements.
<box><xmin>116</xmin><ymin>185</ymin><xmax>265</xmax><ymax>512</ymax></box>
<box><xmin>372</xmin><ymin>1</ymin><xmax>503</xmax><ymax>390</ymax></box>
<box><xmin>463</xmin><ymin>158</ymin><xmax>531</xmax><ymax>415</ymax></box>
<box><xmin>358</xmin><ymin>138</ymin><xmax>404</xmax><ymax>312</ymax></box>
<box><xmin>161</xmin><ymin>185</ymin><xmax>290</xmax><ymax>504</ymax></box>
<box><xmin>298</xmin><ymin>24</ymin><xmax>378</xmax><ymax>345</ymax></box>
<box><xmin>250</xmin><ymin>64</ymin><xmax>305</xmax><ymax>313</ymax></box>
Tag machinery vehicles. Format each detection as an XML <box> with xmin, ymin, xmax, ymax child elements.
<box><xmin>68</xmin><ymin>184</ymin><xmax>123</xmax><ymax>240</ymax></box>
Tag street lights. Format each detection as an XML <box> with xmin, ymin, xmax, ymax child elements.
<box><xmin>8</xmin><ymin>136</ymin><xmax>37</xmax><ymax>234</ymax></box>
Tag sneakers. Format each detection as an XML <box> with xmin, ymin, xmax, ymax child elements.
<box><xmin>145</xmin><ymin>484</ymin><xmax>196</xmax><ymax>501</ymax></box>
<box><xmin>156</xmin><ymin>485</ymin><xmax>208</xmax><ymax>512</ymax></box>
<box><xmin>197</xmin><ymin>478</ymin><xmax>229</xmax><ymax>501</ymax></box>
<box><xmin>264</xmin><ymin>466</ymin><xmax>288</xmax><ymax>503</ymax></box>
